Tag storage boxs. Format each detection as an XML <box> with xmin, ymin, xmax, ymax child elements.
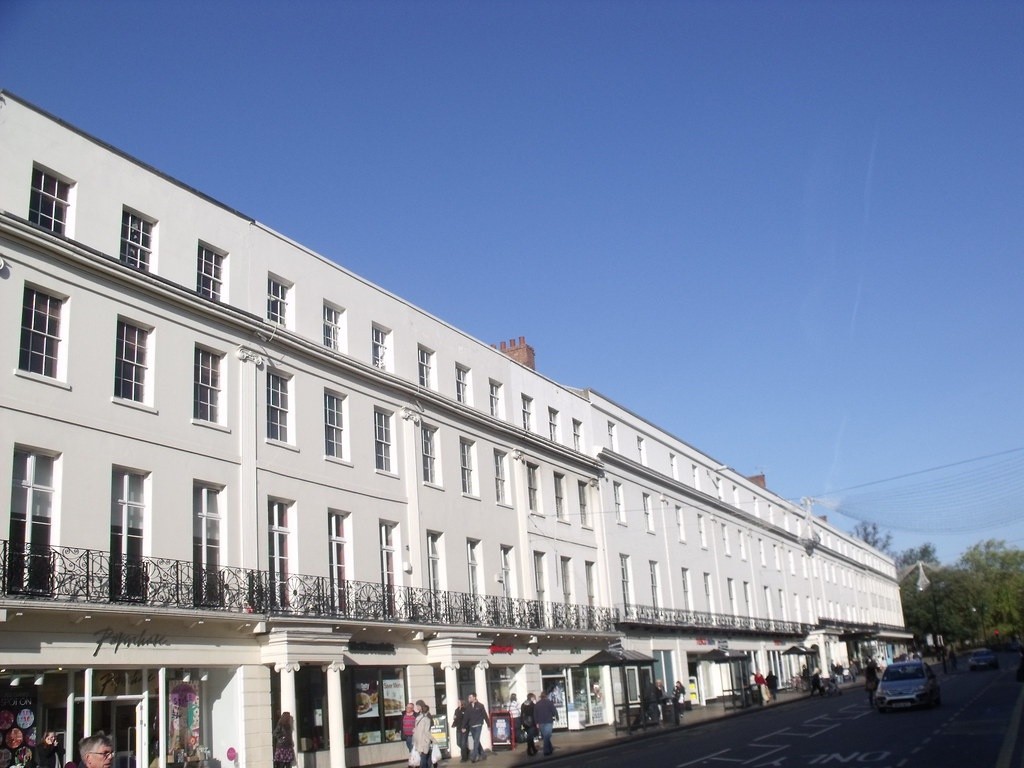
<box><xmin>187</xmin><ymin>746</ymin><xmax>211</xmax><ymax>762</ymax></box>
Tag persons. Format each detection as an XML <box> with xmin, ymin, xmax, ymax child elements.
<box><xmin>64</xmin><ymin>735</ymin><xmax>117</xmax><ymax>768</ymax></box>
<box><xmin>461</xmin><ymin>692</ymin><xmax>491</xmax><ymax>762</ymax></box>
<box><xmin>272</xmin><ymin>712</ymin><xmax>295</xmax><ymax>768</ymax></box>
<box><xmin>394</xmin><ymin>699</ymin><xmax>438</xmax><ymax>768</ymax></box>
<box><xmin>36</xmin><ymin>730</ymin><xmax>65</xmax><ymax>768</ymax></box>
<box><xmin>672</xmin><ymin>681</ymin><xmax>685</xmax><ymax>717</ymax></box>
<box><xmin>831</xmin><ymin>656</ymin><xmax>880</xmax><ymax>705</ymax></box>
<box><xmin>810</xmin><ymin>667</ymin><xmax>823</xmax><ymax>697</ymax></box>
<box><xmin>451</xmin><ymin>698</ymin><xmax>470</xmax><ymax>763</ymax></box>
<box><xmin>508</xmin><ymin>692</ymin><xmax>559</xmax><ymax>756</ymax></box>
<box><xmin>755</xmin><ymin>670</ymin><xmax>778</xmax><ymax>700</ymax></box>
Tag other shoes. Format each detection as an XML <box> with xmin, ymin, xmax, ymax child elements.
<box><xmin>461</xmin><ymin>758</ymin><xmax>468</xmax><ymax>762</ymax></box>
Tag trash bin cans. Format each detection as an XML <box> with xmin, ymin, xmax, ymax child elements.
<box><xmin>661</xmin><ymin>697</ymin><xmax>679</xmax><ymax>726</ymax></box>
<box><xmin>750</xmin><ymin>684</ymin><xmax>762</xmax><ymax>705</ymax></box>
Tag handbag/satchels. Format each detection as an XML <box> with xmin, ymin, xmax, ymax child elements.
<box><xmin>867</xmin><ymin>681</ymin><xmax>877</xmax><ymax>689</ymax></box>
<box><xmin>408</xmin><ymin>746</ymin><xmax>421</xmax><ymax>767</ymax></box>
<box><xmin>432</xmin><ymin>744</ymin><xmax>442</xmax><ymax>764</ymax></box>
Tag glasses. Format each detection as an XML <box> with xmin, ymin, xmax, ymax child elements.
<box><xmin>88</xmin><ymin>751</ymin><xmax>115</xmax><ymax>758</ymax></box>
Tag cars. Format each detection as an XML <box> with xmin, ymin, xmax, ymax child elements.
<box><xmin>968</xmin><ymin>650</ymin><xmax>998</xmax><ymax>672</ymax></box>
<box><xmin>873</xmin><ymin>660</ymin><xmax>941</xmax><ymax>711</ymax></box>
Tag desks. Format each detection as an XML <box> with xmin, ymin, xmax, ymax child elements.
<box><xmin>799</xmin><ymin>675</ymin><xmax>816</xmax><ymax>691</ymax></box>
<box><xmin>610</xmin><ymin>697</ymin><xmax>676</xmax><ymax>734</ymax></box>
<box><xmin>723</xmin><ymin>685</ymin><xmax>753</xmax><ymax>708</ymax></box>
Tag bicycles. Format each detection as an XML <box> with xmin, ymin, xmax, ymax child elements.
<box><xmin>785</xmin><ymin>674</ymin><xmax>842</xmax><ymax>697</ymax></box>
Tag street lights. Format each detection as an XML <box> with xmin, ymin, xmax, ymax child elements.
<box><xmin>920</xmin><ymin>577</ymin><xmax>948</xmax><ymax>674</ymax></box>
